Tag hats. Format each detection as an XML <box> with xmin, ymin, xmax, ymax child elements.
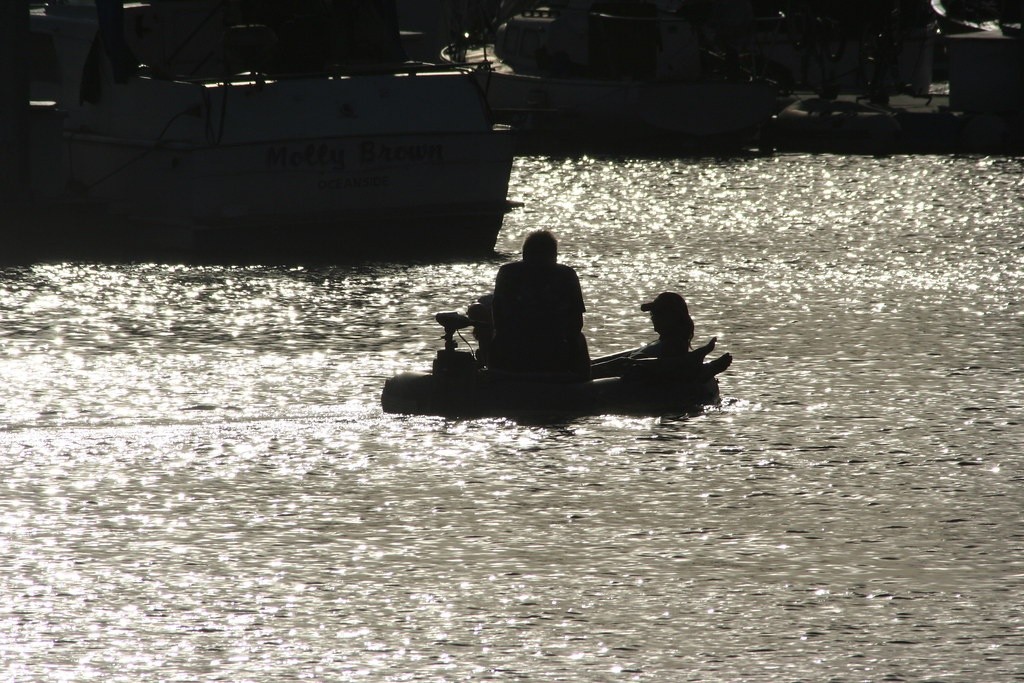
<box><xmin>640</xmin><ymin>292</ymin><xmax>688</xmax><ymax>317</ymax></box>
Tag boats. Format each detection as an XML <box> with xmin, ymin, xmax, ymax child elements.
<box><xmin>381</xmin><ymin>303</ymin><xmax>720</xmax><ymax>416</ymax></box>
<box><xmin>765</xmin><ymin>95</ymin><xmax>900</xmax><ymax>149</ymax></box>
<box><xmin>58</xmin><ymin>22</ymin><xmax>519</xmax><ymax>252</ymax></box>
<box><xmin>438</xmin><ymin>0</ymin><xmax>786</xmax><ymax>151</ymax></box>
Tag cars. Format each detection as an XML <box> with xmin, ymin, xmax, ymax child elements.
<box><xmin>697</xmin><ymin>47</ymin><xmax>780</xmax><ymax>94</ymax></box>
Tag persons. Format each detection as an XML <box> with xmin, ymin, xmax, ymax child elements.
<box><xmin>465</xmin><ymin>231</ymin><xmax>695</xmax><ymax>377</ymax></box>
